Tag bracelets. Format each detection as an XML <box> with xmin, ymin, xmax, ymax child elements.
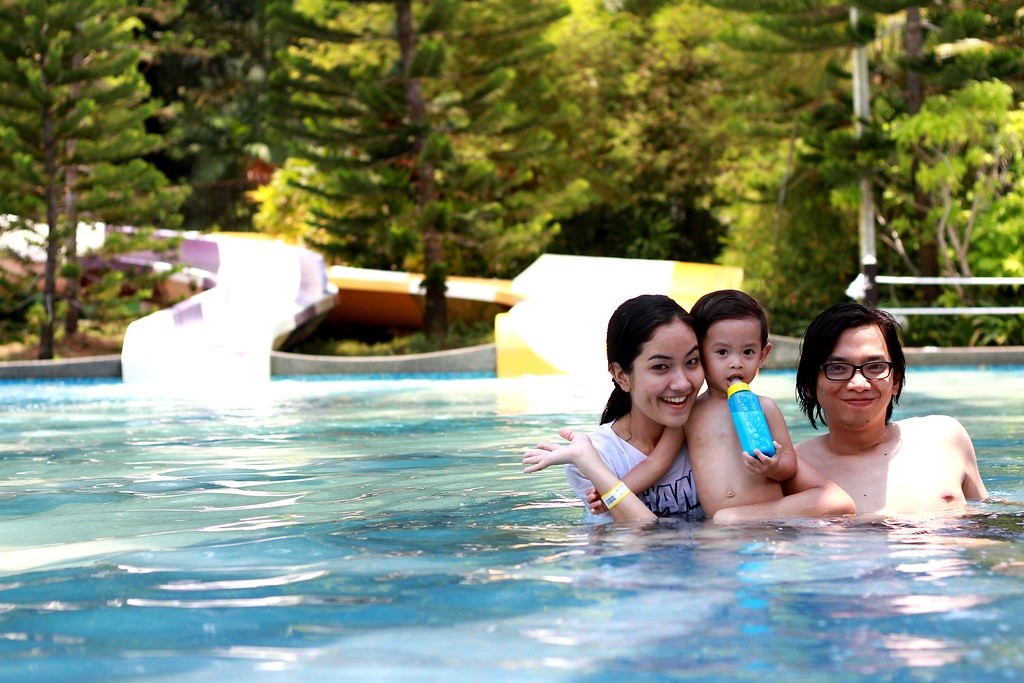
<box><xmin>600</xmin><ymin>480</ymin><xmax>631</xmax><ymax>509</ymax></box>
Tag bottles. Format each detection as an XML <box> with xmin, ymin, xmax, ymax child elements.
<box><xmin>726</xmin><ymin>377</ymin><xmax>776</xmax><ymax>460</ymax></box>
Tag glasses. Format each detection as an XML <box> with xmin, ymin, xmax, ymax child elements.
<box><xmin>818</xmin><ymin>360</ymin><xmax>898</xmax><ymax>382</ymax></box>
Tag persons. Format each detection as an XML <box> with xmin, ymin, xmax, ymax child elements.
<box><xmin>794</xmin><ymin>302</ymin><xmax>989</xmax><ymax>517</ymax></box>
<box><xmin>523</xmin><ymin>289</ymin><xmax>857</xmax><ymax>525</ymax></box>
